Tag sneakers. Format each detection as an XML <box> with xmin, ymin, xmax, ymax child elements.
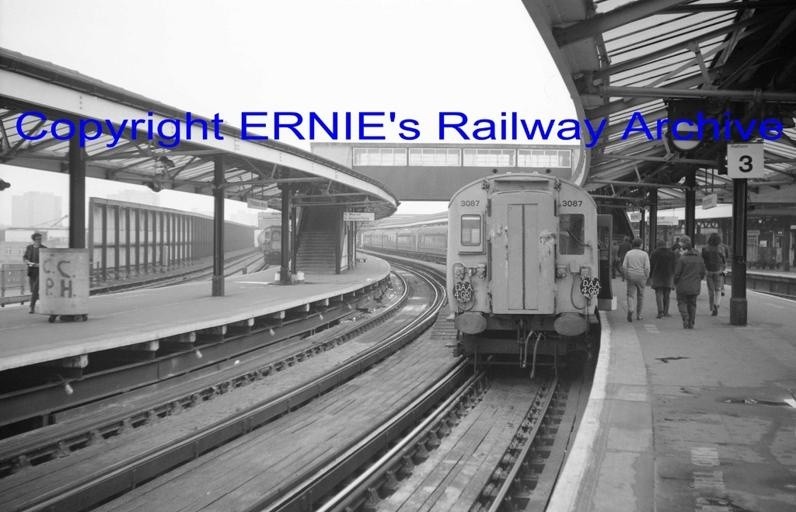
<box><xmin>627</xmin><ymin>305</ymin><xmax>721</xmax><ymax>329</ymax></box>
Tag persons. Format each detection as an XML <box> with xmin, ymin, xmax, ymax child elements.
<box><xmin>23</xmin><ymin>233</ymin><xmax>48</xmax><ymax>313</ymax></box>
<box><xmin>612</xmin><ymin>232</ymin><xmax>728</xmax><ymax>329</ymax></box>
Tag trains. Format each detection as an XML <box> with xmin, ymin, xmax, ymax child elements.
<box><xmin>258</xmin><ymin>225</ymin><xmax>290</xmax><ymax>266</ymax></box>
<box><xmin>360</xmin><ymin>171</ymin><xmax>603</xmax><ymax>375</ymax></box>
